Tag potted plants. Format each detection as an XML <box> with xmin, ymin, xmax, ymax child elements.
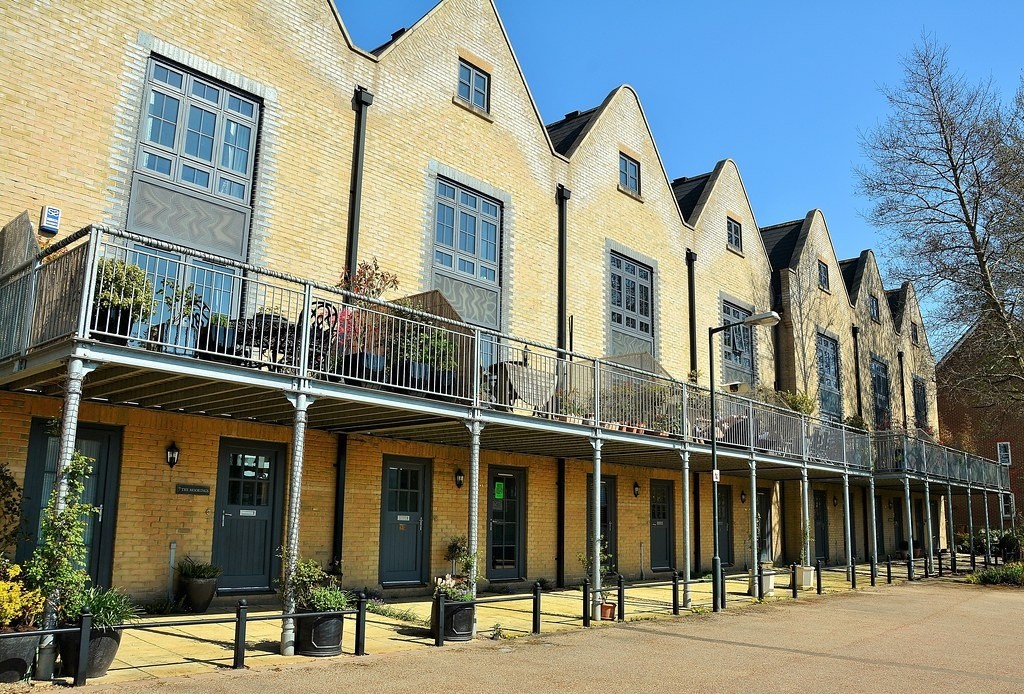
<box><xmin>22</xmin><ymin>447</ymin><xmax>99</xmax><ymax>681</ymax></box>
<box><xmin>55</xmin><ymin>584</ymin><xmax>146</xmax><ymax>677</ymax></box>
<box><xmin>272</xmin><ymin>546</ymin><xmax>357</xmax><ymax>657</ymax></box>
<box><xmin>749</xmin><ymin>512</ymin><xmax>776</xmax><ymax>597</ymax></box>
<box><xmin>601</xmin><ymin>401</ymin><xmax>622</xmax><ymax>429</ymax></box>
<box><xmin>70</xmin><ymin>256</ymin><xmax>159</xmax><ymax>346</ymax></box>
<box><xmin>651</xmin><ymin>413</ymin><xmax>679</xmax><ymax>437</ymax></box>
<box><xmin>429</xmin><ymin>325</ymin><xmax>458</xmax><ymax>403</ymax></box>
<box><xmin>373</xmin><ymin>293</ymin><xmax>435</xmax><ymax>397</ymax></box>
<box><xmin>638</xmin><ymin>419</ymin><xmax>648</xmax><ymax>435</ymax></box>
<box><xmin>559</xmin><ymin>402</ymin><xmax>586</xmax><ymax>424</ymax></box>
<box><xmin>576</xmin><ymin>535</ymin><xmax>617</xmax><ymax>621</ymax></box>
<box><xmin>789</xmin><ymin>520</ymin><xmax>815</xmax><ymax>587</ymax></box>
<box><xmin>898</xmin><ymin>540</ymin><xmax>909</xmax><ymax>560</ymax></box>
<box><xmin>170</xmin><ymin>555</ymin><xmax>225</xmax><ymax>613</ymax></box>
<box><xmin>336</xmin><ymin>256</ymin><xmax>399</xmax><ymax>389</ymax></box>
<box><xmin>626</xmin><ymin>411</ymin><xmax>640</xmax><ymax>433</ymax></box>
<box><xmin>254</xmin><ymin>306</ymin><xmax>288</xmax><ymax>321</ymax></box>
<box><xmin>913</xmin><ymin>540</ymin><xmax>922</xmax><ymax>558</ymax></box>
<box><xmin>198</xmin><ymin>312</ymin><xmax>237</xmax><ymax>365</ymax></box>
<box><xmin>953</xmin><ymin>531</ymin><xmax>970</xmax><ymax>553</ymax></box>
<box><xmin>147</xmin><ymin>278</ymin><xmax>202</xmax><ymax>356</ymax></box>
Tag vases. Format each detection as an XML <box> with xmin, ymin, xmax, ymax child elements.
<box><xmin>0</xmin><ymin>627</ymin><xmax>42</xmax><ymax>683</ymax></box>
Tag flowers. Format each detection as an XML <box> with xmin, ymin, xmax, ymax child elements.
<box><xmin>433</xmin><ymin>574</ymin><xmax>473</xmax><ymax>603</ymax></box>
<box><xmin>0</xmin><ymin>551</ymin><xmax>46</xmax><ymax>633</ymax></box>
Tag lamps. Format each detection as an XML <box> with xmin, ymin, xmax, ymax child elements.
<box><xmin>833</xmin><ymin>496</ymin><xmax>838</xmax><ymax>507</ymax></box>
<box><xmin>166</xmin><ymin>440</ymin><xmax>179</xmax><ymax>469</ymax></box>
<box><xmin>741</xmin><ymin>490</ymin><xmax>746</xmax><ymax>503</ymax></box>
<box><xmin>521</xmin><ymin>345</ymin><xmax>532</xmax><ymax>362</ymax></box>
<box><xmin>888</xmin><ymin>500</ymin><xmax>893</xmax><ymax>510</ymax></box>
<box><xmin>455</xmin><ymin>468</ymin><xmax>465</xmax><ymax>488</ymax></box>
<box><xmin>633</xmin><ymin>482</ymin><xmax>640</xmax><ymax>497</ymax></box>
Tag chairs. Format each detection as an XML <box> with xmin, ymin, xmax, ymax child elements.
<box><xmin>806</xmin><ymin>427</ymin><xmax>834</xmax><ymax>464</ymax></box>
<box><xmin>277</xmin><ymin>300</ymin><xmax>338</xmax><ymax>381</ymax></box>
<box><xmin>189</xmin><ymin>300</ymin><xmax>249</xmax><ymax>367</ymax></box>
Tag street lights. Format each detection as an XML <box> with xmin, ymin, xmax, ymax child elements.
<box><xmin>707</xmin><ymin>310</ymin><xmax>781</xmax><ymax>611</ymax></box>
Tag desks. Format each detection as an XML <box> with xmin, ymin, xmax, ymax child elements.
<box><xmin>230</xmin><ymin>319</ymin><xmax>329</xmax><ymax>371</ymax></box>
<box><xmin>489</xmin><ymin>360</ymin><xmax>528</xmax><ymax>412</ymax></box>
